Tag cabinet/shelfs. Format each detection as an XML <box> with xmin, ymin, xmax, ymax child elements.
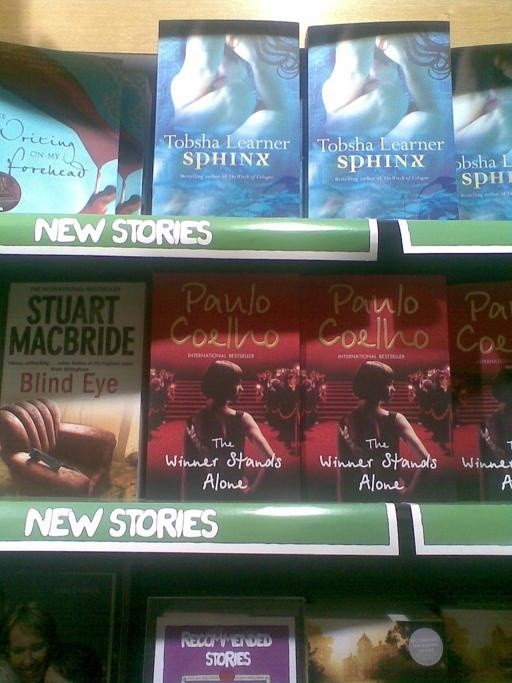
<box><xmin>0</xmin><ymin>211</ymin><xmax>512</xmax><ymax>560</ymax></box>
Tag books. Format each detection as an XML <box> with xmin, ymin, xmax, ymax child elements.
<box><xmin>0</xmin><ymin>568</ymin><xmax>510</xmax><ymax>683</ymax></box>
<box><xmin>0</xmin><ymin>19</ymin><xmax>511</xmax><ymax>221</ymax></box>
<box><xmin>0</xmin><ymin>272</ymin><xmax>510</xmax><ymax>505</ymax></box>
